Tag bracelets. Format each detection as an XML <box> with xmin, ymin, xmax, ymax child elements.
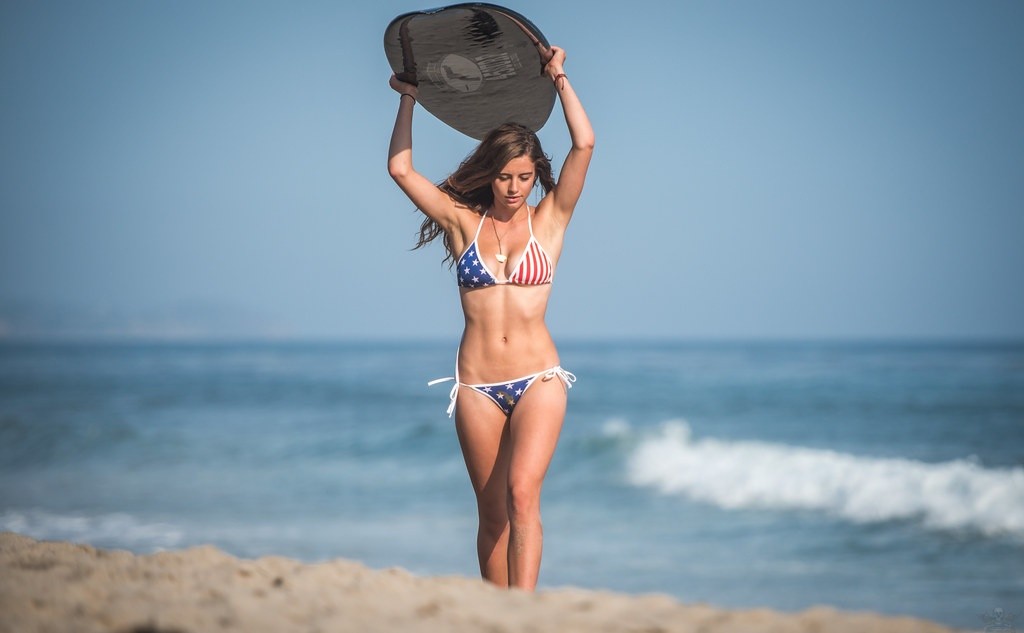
<box><xmin>401</xmin><ymin>94</ymin><xmax>416</xmax><ymax>104</ymax></box>
<box><xmin>553</xmin><ymin>74</ymin><xmax>568</xmax><ymax>90</ymax></box>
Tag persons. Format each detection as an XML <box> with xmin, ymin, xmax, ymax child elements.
<box><xmin>387</xmin><ymin>45</ymin><xmax>595</xmax><ymax>591</ymax></box>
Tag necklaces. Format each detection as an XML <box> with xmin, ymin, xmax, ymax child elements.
<box><xmin>491</xmin><ymin>216</ymin><xmax>508</xmax><ymax>263</ymax></box>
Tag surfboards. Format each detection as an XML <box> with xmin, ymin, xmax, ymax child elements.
<box><xmin>382</xmin><ymin>1</ymin><xmax>559</xmax><ymax>142</ymax></box>
